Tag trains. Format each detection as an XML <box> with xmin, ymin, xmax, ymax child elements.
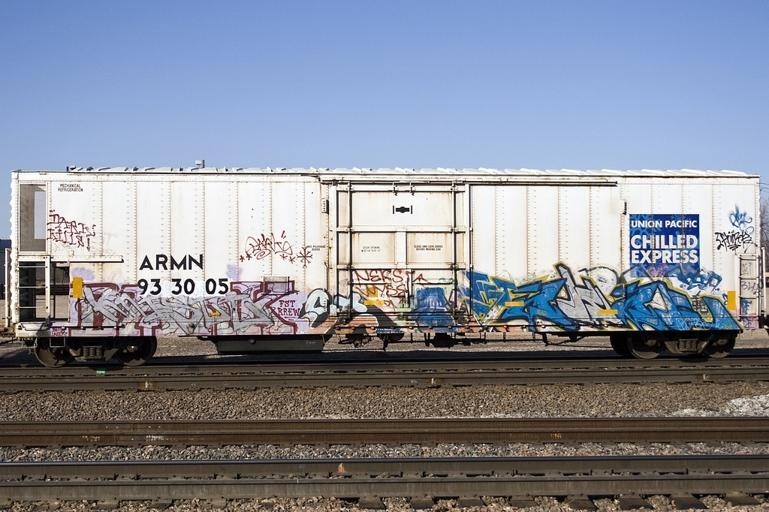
<box><xmin>2</xmin><ymin>161</ymin><xmax>769</xmax><ymax>364</ymax></box>
